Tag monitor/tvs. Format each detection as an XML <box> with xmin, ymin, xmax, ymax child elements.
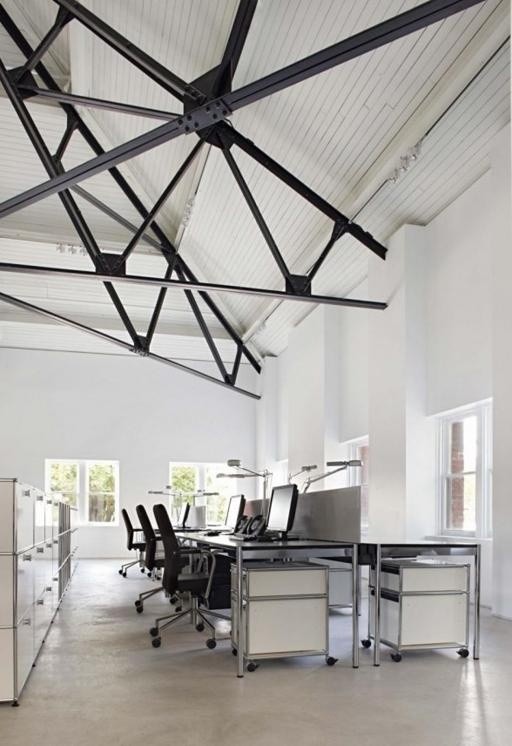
<box><xmin>265</xmin><ymin>484</ymin><xmax>299</xmax><ymax>541</ymax></box>
<box><xmin>179</xmin><ymin>502</ymin><xmax>190</xmax><ymax>528</ymax></box>
<box><xmin>222</xmin><ymin>494</ymin><xmax>246</xmax><ymax>535</ymax></box>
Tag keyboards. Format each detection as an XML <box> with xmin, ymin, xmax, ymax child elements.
<box><xmin>229</xmin><ymin>534</ymin><xmax>257</xmax><ymax>541</ymax></box>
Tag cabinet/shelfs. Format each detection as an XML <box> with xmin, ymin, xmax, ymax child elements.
<box><xmin>229</xmin><ymin>559</ymin><xmax>332</xmax><ymax>659</ymax></box>
<box><xmin>0</xmin><ymin>477</ymin><xmax>79</xmax><ymax>707</ymax></box>
<box><xmin>369</xmin><ymin>557</ymin><xmax>472</xmax><ymax>650</ymax></box>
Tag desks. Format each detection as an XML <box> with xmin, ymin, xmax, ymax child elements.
<box><xmin>364</xmin><ymin>539</ymin><xmax>484</xmax><ymax>668</ymax></box>
<box><xmin>174</xmin><ymin>531</ymin><xmax>364</xmax><ymax>678</ymax></box>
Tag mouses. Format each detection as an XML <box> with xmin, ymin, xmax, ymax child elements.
<box><xmin>208</xmin><ymin>532</ymin><xmax>219</xmax><ymax>536</ymax></box>
<box><xmin>259</xmin><ymin>536</ymin><xmax>272</xmax><ymax>542</ymax></box>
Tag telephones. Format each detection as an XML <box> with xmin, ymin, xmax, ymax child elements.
<box><xmin>234</xmin><ymin>515</ymin><xmax>267</xmax><ymax>539</ymax></box>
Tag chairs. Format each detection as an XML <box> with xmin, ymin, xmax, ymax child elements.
<box><xmin>118</xmin><ymin>503</ymin><xmax>234</xmax><ymax>650</ymax></box>
